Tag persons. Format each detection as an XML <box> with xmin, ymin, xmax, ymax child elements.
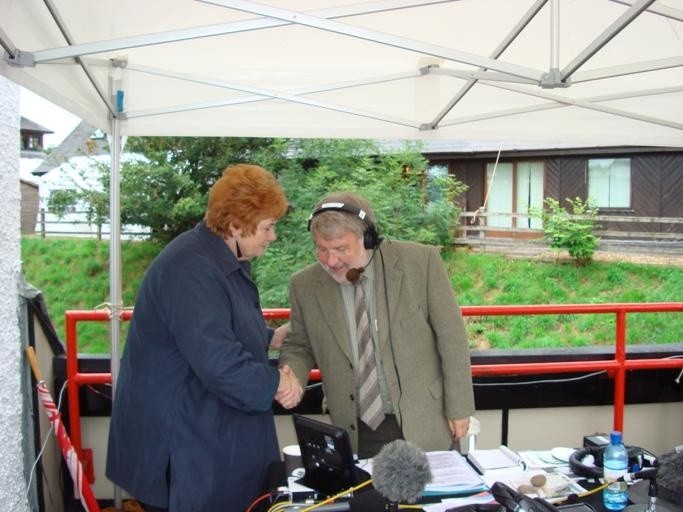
<box><xmin>273</xmin><ymin>189</ymin><xmax>478</xmax><ymax>460</ymax></box>
<box><xmin>103</xmin><ymin>162</ymin><xmax>305</xmax><ymax>511</ymax></box>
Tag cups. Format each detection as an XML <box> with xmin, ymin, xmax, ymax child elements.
<box><xmin>283</xmin><ymin>445</ymin><xmax>307</xmax><ymax>485</ymax></box>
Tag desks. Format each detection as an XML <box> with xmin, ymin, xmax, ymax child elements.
<box><xmin>269</xmin><ymin>447</ymin><xmax>683</xmax><ymax>512</ymax></box>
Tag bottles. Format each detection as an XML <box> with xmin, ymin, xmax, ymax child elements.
<box><xmin>604</xmin><ymin>430</ymin><xmax>628</xmax><ymax>509</ymax></box>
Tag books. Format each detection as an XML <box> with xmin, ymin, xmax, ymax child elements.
<box><xmin>466</xmin><ymin>445</ymin><xmax>526</xmax><ymax>475</ymax></box>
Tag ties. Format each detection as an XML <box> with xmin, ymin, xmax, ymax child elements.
<box><xmin>359</xmin><ymin>277</ymin><xmax>385</xmax><ymax>432</ymax></box>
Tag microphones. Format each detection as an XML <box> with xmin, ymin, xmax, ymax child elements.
<box><xmin>345</xmin><ymin>238</ymin><xmax>384</xmax><ymax>282</ymax></box>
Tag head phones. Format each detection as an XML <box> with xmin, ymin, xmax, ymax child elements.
<box><xmin>306</xmin><ymin>202</ymin><xmax>379</xmax><ymax>250</ymax></box>
